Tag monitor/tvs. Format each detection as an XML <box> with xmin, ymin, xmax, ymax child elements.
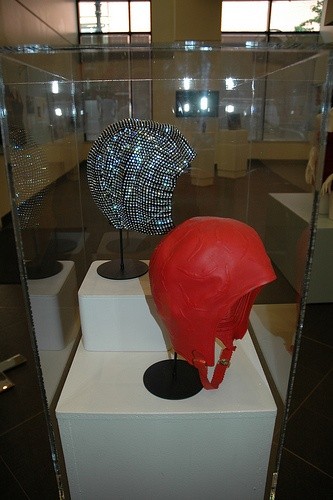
<box><xmin>175</xmin><ymin>90</ymin><xmax>219</xmax><ymax>117</ymax></box>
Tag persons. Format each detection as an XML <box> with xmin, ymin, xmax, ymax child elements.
<box><xmin>305</xmin><ymin>106</ymin><xmax>333</xmax><ymax>221</ymax></box>
